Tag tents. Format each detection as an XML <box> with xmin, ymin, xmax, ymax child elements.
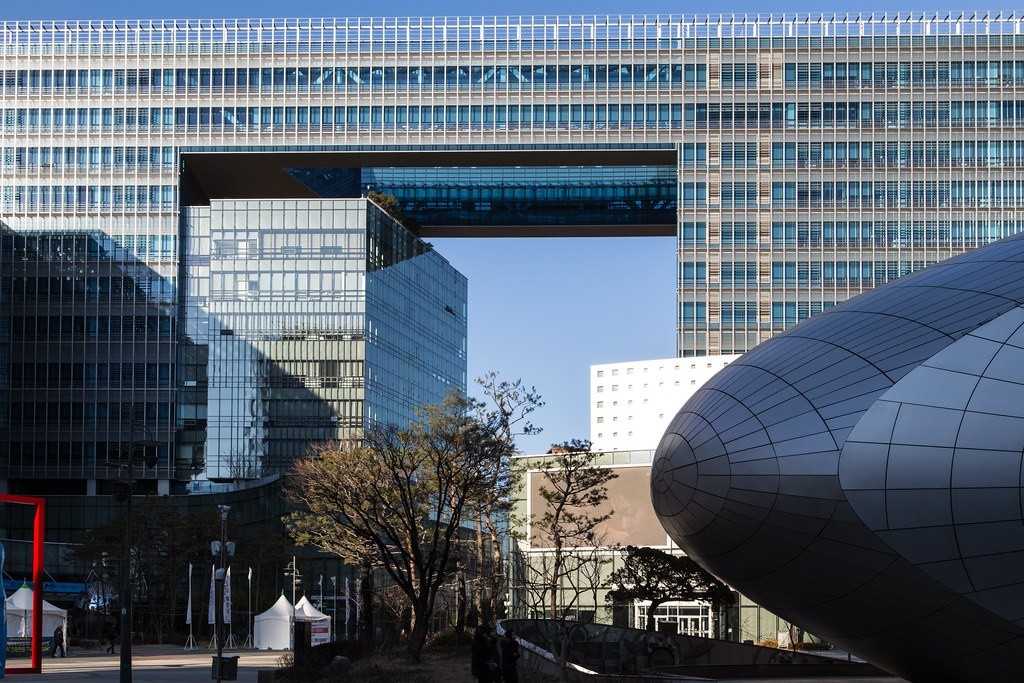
<box><xmin>4</xmin><ymin>577</ymin><xmax>69</xmax><ymax>656</ymax></box>
<box><xmin>253</xmin><ymin>589</ymin><xmax>331</xmax><ymax>651</ymax></box>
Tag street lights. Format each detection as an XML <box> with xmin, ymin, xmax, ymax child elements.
<box><xmin>283</xmin><ymin>555</ymin><xmax>296</xmax><ymax>649</ymax></box>
<box><xmin>120</xmin><ymin>405</ymin><xmax>159</xmax><ymax>683</ymax></box>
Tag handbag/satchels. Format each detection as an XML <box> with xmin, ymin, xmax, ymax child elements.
<box><xmin>486</xmin><ymin>658</ymin><xmax>498</xmax><ymax>671</ymax></box>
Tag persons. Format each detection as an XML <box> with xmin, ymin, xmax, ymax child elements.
<box><xmin>51</xmin><ymin>625</ymin><xmax>67</xmax><ymax>658</ymax></box>
<box><xmin>107</xmin><ymin>622</ymin><xmax>121</xmax><ymax>654</ymax></box>
<box><xmin>471</xmin><ymin>624</ymin><xmax>520</xmax><ymax>683</ymax></box>
